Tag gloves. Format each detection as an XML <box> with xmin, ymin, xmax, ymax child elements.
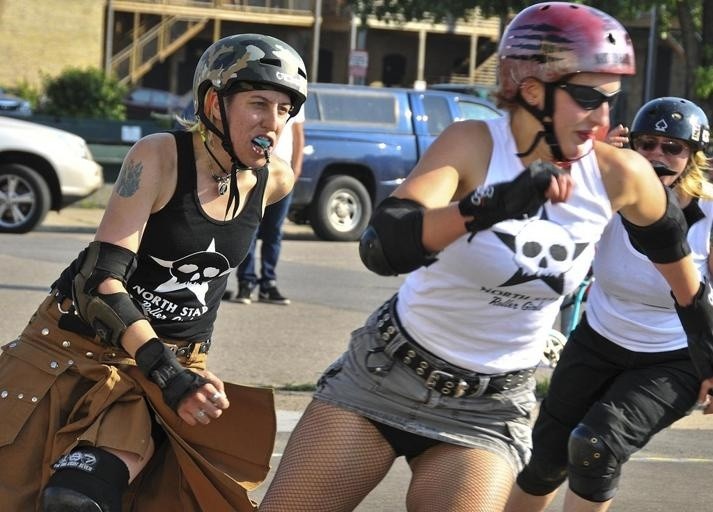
<box><xmin>460</xmin><ymin>159</ymin><xmax>564</xmax><ymax>233</ymax></box>
<box><xmin>133</xmin><ymin>338</ymin><xmax>209</xmax><ymax>419</ymax></box>
<box><xmin>677</xmin><ymin>287</ymin><xmax>713</xmax><ymax>397</ymax></box>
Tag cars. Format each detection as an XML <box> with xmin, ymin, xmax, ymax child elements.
<box><xmin>0</xmin><ymin>92</ymin><xmax>32</xmax><ymax>116</ymax></box>
<box><xmin>120</xmin><ymin>89</ymin><xmax>190</xmax><ymax>121</ymax></box>
<box><xmin>1</xmin><ymin>116</ymin><xmax>104</xmax><ymax>234</ymax></box>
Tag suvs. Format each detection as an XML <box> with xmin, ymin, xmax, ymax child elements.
<box><xmin>177</xmin><ymin>83</ymin><xmax>503</xmax><ymax>241</ymax></box>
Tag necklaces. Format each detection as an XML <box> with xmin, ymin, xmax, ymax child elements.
<box><xmin>201</xmin><ymin>131</ymin><xmax>241</xmax><ymax>196</ymax></box>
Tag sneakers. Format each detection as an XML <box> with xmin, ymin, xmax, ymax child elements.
<box><xmin>259</xmin><ymin>287</ymin><xmax>290</xmax><ymax>305</ymax></box>
<box><xmin>236</xmin><ymin>284</ymin><xmax>251</xmax><ymax>304</ymax></box>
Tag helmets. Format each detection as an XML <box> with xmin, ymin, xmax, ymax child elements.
<box><xmin>628</xmin><ymin>97</ymin><xmax>711</xmax><ymax>149</ymax></box>
<box><xmin>496</xmin><ymin>2</ymin><xmax>634</xmax><ymax>101</ymax></box>
<box><xmin>193</xmin><ymin>33</ymin><xmax>307</xmax><ymax>114</ymax></box>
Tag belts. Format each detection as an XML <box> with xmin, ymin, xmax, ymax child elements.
<box><xmin>378</xmin><ymin>295</ymin><xmax>540</xmax><ymax>398</ymax></box>
<box><xmin>51</xmin><ymin>278</ymin><xmax>211</xmax><ymax>359</ymax></box>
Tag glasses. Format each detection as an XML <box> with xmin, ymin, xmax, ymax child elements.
<box><xmin>554</xmin><ymin>81</ymin><xmax>621</xmax><ymax>112</ymax></box>
<box><xmin>638</xmin><ymin>136</ymin><xmax>692</xmax><ymax>155</ymax></box>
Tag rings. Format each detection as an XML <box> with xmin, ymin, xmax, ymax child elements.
<box><xmin>209</xmin><ymin>392</ymin><xmax>222</xmax><ymax>403</ymax></box>
<box><xmin>195</xmin><ymin>410</ymin><xmax>205</xmax><ymax>419</ymax></box>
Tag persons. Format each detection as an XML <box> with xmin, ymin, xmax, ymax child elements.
<box><xmin>508</xmin><ymin>97</ymin><xmax>711</xmax><ymax>511</ymax></box>
<box><xmin>0</xmin><ymin>33</ymin><xmax>307</xmax><ymax>512</ymax></box>
<box><xmin>259</xmin><ymin>1</ymin><xmax>712</xmax><ymax>512</ymax></box>
<box><xmin>234</xmin><ymin>101</ymin><xmax>305</xmax><ymax>306</ymax></box>
